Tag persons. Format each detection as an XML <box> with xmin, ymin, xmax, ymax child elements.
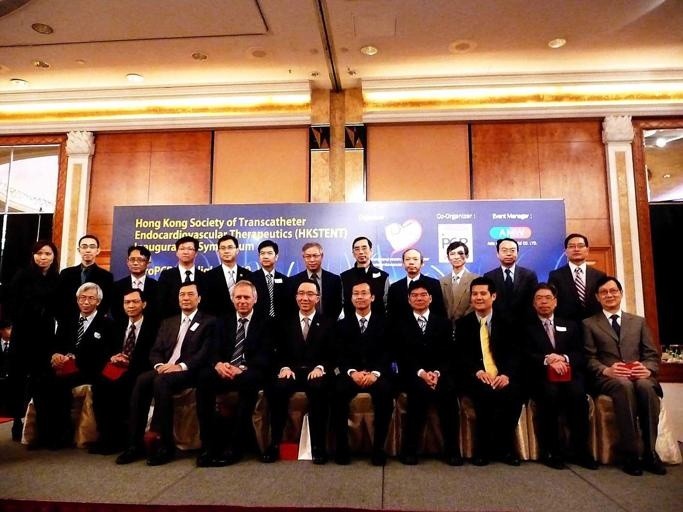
<box><xmin>119</xmin><ymin>281</ymin><xmax>216</xmax><ymax>466</ymax></box>
<box><xmin>157</xmin><ymin>235</ymin><xmax>208</xmax><ymax>315</ymax></box>
<box><xmin>543</xmin><ymin>233</ymin><xmax>606</xmax><ymax>317</ymax></box>
<box><xmin>331</xmin><ymin>282</ymin><xmax>403</xmax><ymax>467</ymax></box>
<box><xmin>440</xmin><ymin>242</ymin><xmax>481</xmax><ymax>324</ymax></box>
<box><xmin>6</xmin><ymin>242</ymin><xmax>60</xmax><ymax>440</ymax></box>
<box><xmin>521</xmin><ymin>282</ymin><xmax>606</xmax><ymax>467</ymax></box>
<box><xmin>30</xmin><ymin>280</ymin><xmax>118</xmax><ymax>452</ymax></box>
<box><xmin>388</xmin><ymin>248</ymin><xmax>441</xmax><ymax>314</ymax></box>
<box><xmin>284</xmin><ymin>242</ymin><xmax>341</xmax><ymax>324</ymax></box>
<box><xmin>86</xmin><ymin>291</ymin><xmax>159</xmax><ymax>456</ymax></box>
<box><xmin>108</xmin><ymin>245</ymin><xmax>157</xmax><ymax>321</ymax></box>
<box><xmin>482</xmin><ymin>241</ymin><xmax>539</xmax><ymax>332</ymax></box>
<box><xmin>262</xmin><ymin>280</ymin><xmax>340</xmax><ymax>463</ymax></box>
<box><xmin>340</xmin><ymin>237</ymin><xmax>389</xmax><ymax>318</ymax></box>
<box><xmin>204</xmin><ymin>235</ymin><xmax>252</xmax><ymax>314</ymax></box>
<box><xmin>54</xmin><ymin>236</ymin><xmax>107</xmax><ymax>323</ymax></box>
<box><xmin>194</xmin><ymin>280</ymin><xmax>280</xmax><ymax>466</ymax></box>
<box><xmin>395</xmin><ymin>285</ymin><xmax>470</xmax><ymax>464</ymax></box>
<box><xmin>454</xmin><ymin>279</ymin><xmax>536</xmax><ymax>460</ymax></box>
<box><xmin>579</xmin><ymin>277</ymin><xmax>670</xmax><ymax>474</ymax></box>
<box><xmin>246</xmin><ymin>240</ymin><xmax>287</xmax><ymax>324</ymax></box>
<box><xmin>0</xmin><ymin>317</ymin><xmax>17</xmax><ymax>380</ymax></box>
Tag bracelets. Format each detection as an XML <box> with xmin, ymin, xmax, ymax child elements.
<box><xmin>371</xmin><ymin>373</ymin><xmax>379</xmax><ymax>378</ymax></box>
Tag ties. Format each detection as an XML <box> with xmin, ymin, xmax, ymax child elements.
<box><xmin>302</xmin><ymin>273</ymin><xmax>427</xmax><ymax>342</ymax></box>
<box><xmin>74</xmin><ymin>268</ymin><xmax>277</xmax><ymax>367</ymax></box>
<box><xmin>451</xmin><ymin>268</ymin><xmax>619</xmax><ymax>381</ymax></box>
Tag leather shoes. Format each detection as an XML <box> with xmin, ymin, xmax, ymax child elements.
<box><xmin>82</xmin><ymin>437</ymin><xmax>668</xmax><ymax>477</ymax></box>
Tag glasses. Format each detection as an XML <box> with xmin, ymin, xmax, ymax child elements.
<box><xmin>80</xmin><ymin>245</ymin><xmax>97</xmax><ymax>248</ymax></box>
<box><xmin>128</xmin><ymin>257</ymin><xmax>147</xmax><ymax>261</ymax></box>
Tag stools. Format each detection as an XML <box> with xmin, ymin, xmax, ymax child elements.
<box><xmin>527</xmin><ymin>394</ymin><xmax>596</xmax><ymax>460</ymax></box>
<box><xmin>461</xmin><ymin>397</ymin><xmax>529</xmax><ymax>460</ymax></box>
<box><xmin>597</xmin><ymin>394</ymin><xmax>683</xmax><ymax>465</ymax></box>
<box><xmin>396</xmin><ymin>394</ymin><xmax>461</xmax><ymax>460</ymax></box>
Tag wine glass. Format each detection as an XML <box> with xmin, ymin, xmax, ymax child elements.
<box><xmin>669</xmin><ymin>345</ymin><xmax>683</xmax><ymax>362</ymax></box>
